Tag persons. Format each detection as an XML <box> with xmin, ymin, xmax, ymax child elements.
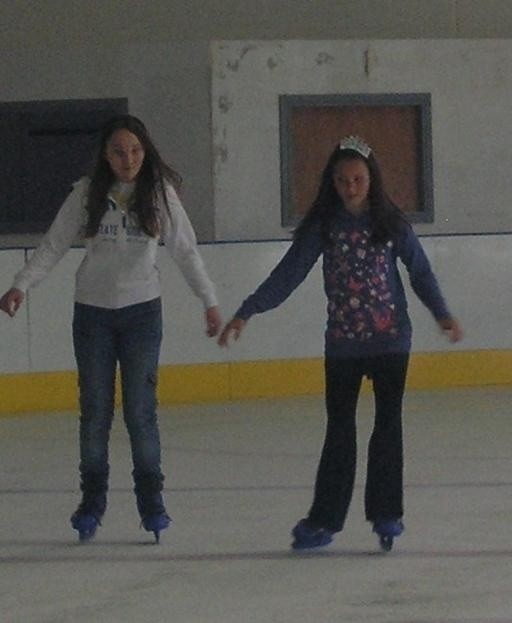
<box><xmin>215</xmin><ymin>130</ymin><xmax>464</xmax><ymax>544</ymax></box>
<box><xmin>1</xmin><ymin>109</ymin><xmax>225</xmax><ymax>536</ymax></box>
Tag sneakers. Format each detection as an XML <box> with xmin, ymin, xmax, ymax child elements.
<box><xmin>70</xmin><ymin>483</ymin><xmax>108</xmax><ymax>532</ymax></box>
<box><xmin>134</xmin><ymin>481</ymin><xmax>171</xmax><ymax>532</ymax></box>
<box><xmin>291</xmin><ymin>518</ymin><xmax>344</xmax><ymax>544</ymax></box>
<box><xmin>372</xmin><ymin>518</ymin><xmax>404</xmax><ymax>536</ymax></box>
